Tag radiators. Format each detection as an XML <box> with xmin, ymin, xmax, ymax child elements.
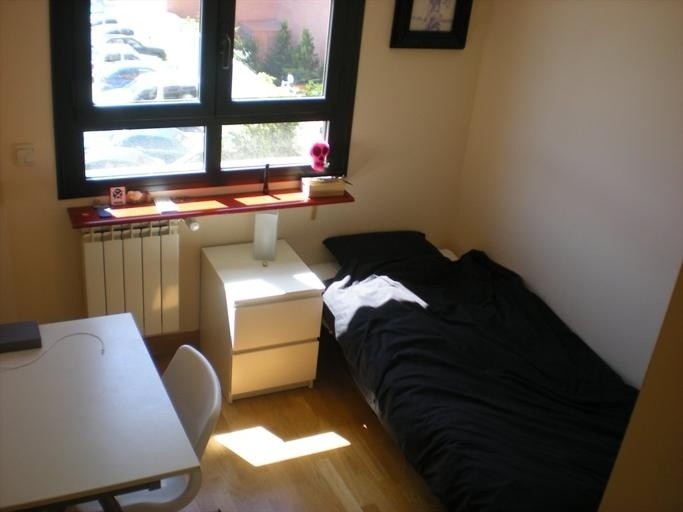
<box><xmin>81</xmin><ymin>225</ymin><xmax>179</xmax><ymax>338</ymax></box>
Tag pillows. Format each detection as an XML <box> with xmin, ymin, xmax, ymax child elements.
<box><xmin>321</xmin><ymin>229</ymin><xmax>451</xmax><ymax>282</ymax></box>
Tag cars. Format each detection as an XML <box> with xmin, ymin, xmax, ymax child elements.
<box><xmin>86</xmin><ymin>15</ymin><xmax>205</xmax><ymax>171</ymax></box>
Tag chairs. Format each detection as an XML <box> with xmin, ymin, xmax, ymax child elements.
<box><xmin>74</xmin><ymin>344</ymin><xmax>222</xmax><ymax>512</ymax></box>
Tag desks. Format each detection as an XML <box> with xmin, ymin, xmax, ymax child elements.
<box><xmin>0</xmin><ymin>312</ymin><xmax>200</xmax><ymax>510</ymax></box>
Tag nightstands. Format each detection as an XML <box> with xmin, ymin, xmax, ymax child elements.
<box><xmin>200</xmin><ymin>238</ymin><xmax>325</xmax><ymax>403</ymax></box>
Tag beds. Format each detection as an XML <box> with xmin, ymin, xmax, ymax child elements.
<box><xmin>307</xmin><ymin>247</ymin><xmax>639</xmax><ymax>509</ymax></box>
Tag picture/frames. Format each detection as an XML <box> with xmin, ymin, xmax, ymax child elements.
<box><xmin>389</xmin><ymin>1</ymin><xmax>473</xmax><ymax>50</ymax></box>
